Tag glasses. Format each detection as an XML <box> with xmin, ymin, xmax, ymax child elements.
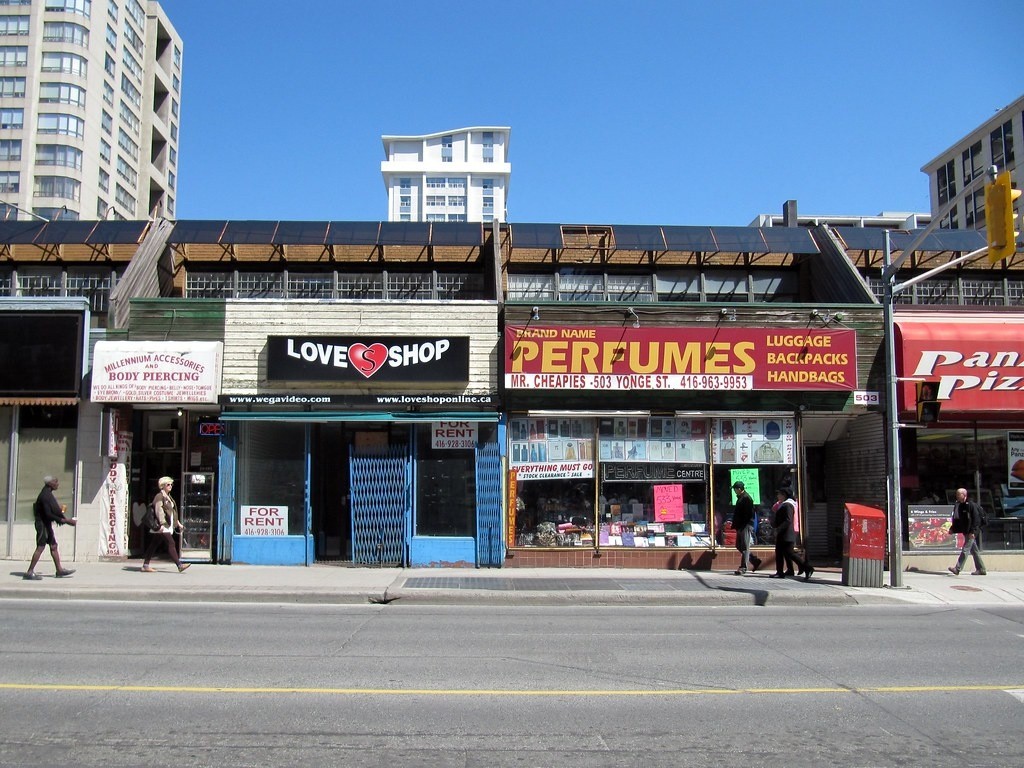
<box><xmin>167</xmin><ymin>484</ymin><xmax>173</xmax><ymax>486</ymax></box>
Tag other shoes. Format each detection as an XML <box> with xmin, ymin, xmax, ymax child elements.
<box><xmin>23</xmin><ymin>573</ymin><xmax>42</xmax><ymax>580</ymax></box>
<box><xmin>752</xmin><ymin>558</ymin><xmax>761</xmax><ymax>572</ymax></box>
<box><xmin>769</xmin><ymin>573</ymin><xmax>785</xmax><ymax>578</ymax></box>
<box><xmin>806</xmin><ymin>569</ymin><xmax>814</xmax><ymax>579</ymax></box>
<box><xmin>784</xmin><ymin>570</ymin><xmax>794</xmax><ymax>575</ymax></box>
<box><xmin>56</xmin><ymin>569</ymin><xmax>76</xmax><ymax>578</ymax></box>
<box><xmin>735</xmin><ymin>568</ymin><xmax>747</xmax><ymax>574</ymax></box>
<box><xmin>141</xmin><ymin>567</ymin><xmax>157</xmax><ymax>572</ymax></box>
<box><xmin>798</xmin><ymin>569</ymin><xmax>805</xmax><ymax>575</ymax></box>
<box><xmin>178</xmin><ymin>563</ymin><xmax>191</xmax><ymax>572</ymax></box>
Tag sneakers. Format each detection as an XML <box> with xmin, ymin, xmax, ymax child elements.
<box><xmin>948</xmin><ymin>567</ymin><xmax>959</xmax><ymax>575</ymax></box>
<box><xmin>971</xmin><ymin>570</ymin><xmax>986</xmax><ymax>575</ymax></box>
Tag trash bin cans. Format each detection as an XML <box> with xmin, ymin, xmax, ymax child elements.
<box><xmin>840</xmin><ymin>502</ymin><xmax>887</xmax><ymax>588</ymax></box>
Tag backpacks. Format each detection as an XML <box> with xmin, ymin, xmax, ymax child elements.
<box><xmin>976</xmin><ymin>504</ymin><xmax>989</xmax><ymax>533</ymax></box>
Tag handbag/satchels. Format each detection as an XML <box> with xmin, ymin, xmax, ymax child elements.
<box><xmin>142</xmin><ymin>492</ymin><xmax>165</xmax><ymax>531</ymax></box>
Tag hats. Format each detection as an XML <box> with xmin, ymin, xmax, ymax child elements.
<box><xmin>731</xmin><ymin>481</ymin><xmax>744</xmax><ymax>488</ymax></box>
<box><xmin>776</xmin><ymin>488</ymin><xmax>791</xmax><ymax>499</ymax></box>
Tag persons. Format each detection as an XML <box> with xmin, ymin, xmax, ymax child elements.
<box><xmin>770</xmin><ymin>487</ymin><xmax>815</xmax><ymax>580</ymax></box>
<box><xmin>140</xmin><ymin>477</ymin><xmax>192</xmax><ymax>573</ymax></box>
<box><xmin>948</xmin><ymin>488</ymin><xmax>988</xmax><ymax>576</ymax></box>
<box><xmin>23</xmin><ymin>475</ymin><xmax>77</xmax><ymax>580</ymax></box>
<box><xmin>731</xmin><ymin>482</ymin><xmax>762</xmax><ymax>575</ymax></box>
